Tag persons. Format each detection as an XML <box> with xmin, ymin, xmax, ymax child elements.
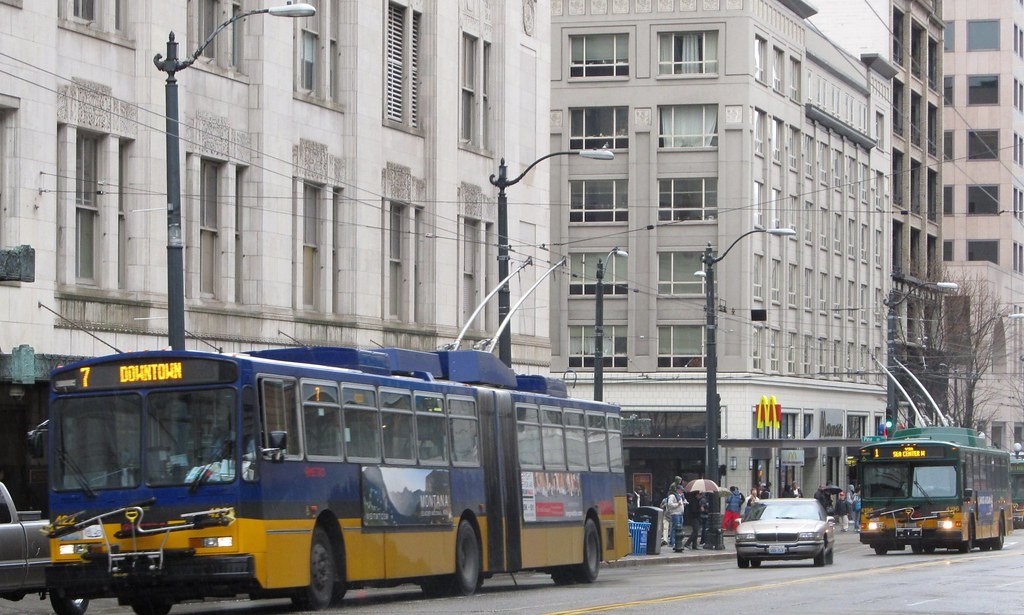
<box><xmin>722</xmin><ymin>486</ymin><xmax>745</xmax><ymax>531</ymax></box>
<box><xmin>211</xmin><ymin>414</ymin><xmax>257</xmax><ymax>463</ymax></box>
<box><xmin>627</xmin><ymin>486</ymin><xmax>647</xmax><ymax>522</ymax></box>
<box><xmin>783</xmin><ymin>481</ymin><xmax>803</xmax><ymax>498</ymax></box>
<box><xmin>761</xmin><ymin>482</ymin><xmax>772</xmax><ymax>499</ymax></box>
<box><xmin>745</xmin><ymin>488</ymin><xmax>760</xmax><ymax>515</ymax></box>
<box><xmin>814</xmin><ymin>485</ymin><xmax>861</xmax><ymax>533</ymax></box>
<box><xmin>660</xmin><ymin>476</ymin><xmax>689</xmax><ymax>547</ymax></box>
<box><xmin>684</xmin><ymin>491</ymin><xmax>710</xmax><ymax>550</ymax></box>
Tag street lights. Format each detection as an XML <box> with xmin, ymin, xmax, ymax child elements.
<box><xmin>489</xmin><ymin>151</ymin><xmax>616</xmax><ymax>371</ymax></box>
<box><xmin>154</xmin><ymin>2</ymin><xmax>317</xmax><ymax>351</ymax></box>
<box><xmin>595</xmin><ymin>248</ymin><xmax>630</xmax><ymax>400</ymax></box>
<box><xmin>701</xmin><ymin>228</ymin><xmax>796</xmax><ymax>547</ymax></box>
<box><xmin>955</xmin><ymin>314</ymin><xmax>1024</xmax><ymax>431</ymax></box>
<box><xmin>1014</xmin><ymin>443</ymin><xmax>1022</xmax><ymax>458</ymax></box>
<box><xmin>883</xmin><ymin>281</ymin><xmax>958</xmax><ymax>437</ymax></box>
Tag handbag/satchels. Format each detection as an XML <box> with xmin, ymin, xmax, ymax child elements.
<box><xmin>854</xmin><ymin>495</ymin><xmax>861</xmax><ymax>511</ymax></box>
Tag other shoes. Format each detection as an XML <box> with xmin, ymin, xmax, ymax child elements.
<box><xmin>684</xmin><ymin>544</ymin><xmax>691</xmax><ymax>550</ymax></box>
<box><xmin>683</xmin><ymin>502</ymin><xmax>689</xmax><ymax>505</ymax></box>
<box><xmin>853</xmin><ymin>528</ymin><xmax>860</xmax><ymax>532</ymax></box>
<box><xmin>840</xmin><ymin>529</ymin><xmax>848</xmax><ymax>532</ymax></box>
<box><xmin>668</xmin><ymin>544</ymin><xmax>675</xmax><ymax>548</ymax></box>
<box><xmin>693</xmin><ymin>547</ymin><xmax>703</xmax><ymax>551</ymax></box>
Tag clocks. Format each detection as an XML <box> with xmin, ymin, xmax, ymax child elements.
<box><xmin>523</xmin><ymin>0</ymin><xmax>535</xmax><ymax>36</ymax></box>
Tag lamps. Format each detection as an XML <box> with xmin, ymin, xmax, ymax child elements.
<box><xmin>776</xmin><ymin>455</ymin><xmax>780</xmax><ymax>468</ymax></box>
<box><xmin>822</xmin><ymin>454</ymin><xmax>827</xmax><ymax>466</ymax></box>
<box><xmin>750</xmin><ymin>457</ymin><xmax>753</xmax><ymax>470</ymax></box>
<box><xmin>730</xmin><ymin>457</ymin><xmax>737</xmax><ymax>470</ymax></box>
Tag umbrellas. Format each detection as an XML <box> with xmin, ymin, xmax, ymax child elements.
<box><xmin>684</xmin><ymin>477</ymin><xmax>719</xmax><ymax>493</ymax></box>
<box><xmin>825</xmin><ymin>485</ymin><xmax>842</xmax><ymax>495</ymax></box>
<box><xmin>716</xmin><ymin>487</ymin><xmax>733</xmax><ymax>498</ymax></box>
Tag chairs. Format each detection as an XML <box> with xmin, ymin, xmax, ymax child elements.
<box><xmin>229</xmin><ymin>430</ymin><xmax>286</xmax><ymax>464</ymax></box>
<box><xmin>312</xmin><ymin>424</ymin><xmax>430</xmax><ymax>461</ymax></box>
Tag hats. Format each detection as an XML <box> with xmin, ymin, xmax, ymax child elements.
<box><xmin>677</xmin><ymin>485</ymin><xmax>684</xmax><ymax>490</ymax></box>
<box><xmin>840</xmin><ymin>491</ymin><xmax>845</xmax><ymax>496</ymax></box>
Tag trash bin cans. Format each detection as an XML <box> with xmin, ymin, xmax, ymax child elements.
<box><xmin>628</xmin><ymin>522</ymin><xmax>652</xmax><ymax>556</ymax></box>
<box><xmin>634</xmin><ymin>506</ymin><xmax>664</xmax><ymax>554</ymax></box>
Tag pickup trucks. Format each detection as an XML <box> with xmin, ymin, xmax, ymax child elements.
<box><xmin>0</xmin><ymin>481</ymin><xmax>51</xmax><ymax>605</ymax></box>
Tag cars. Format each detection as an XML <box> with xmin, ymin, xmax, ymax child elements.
<box><xmin>734</xmin><ymin>499</ymin><xmax>835</xmax><ymax>569</ymax></box>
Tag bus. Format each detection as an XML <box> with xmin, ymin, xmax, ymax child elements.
<box><xmin>24</xmin><ymin>345</ymin><xmax>635</xmax><ymax>615</ymax></box>
<box><xmin>1010</xmin><ymin>459</ymin><xmax>1024</xmax><ymax>529</ymax></box>
<box><xmin>848</xmin><ymin>427</ymin><xmax>1012</xmax><ymax>557</ymax></box>
